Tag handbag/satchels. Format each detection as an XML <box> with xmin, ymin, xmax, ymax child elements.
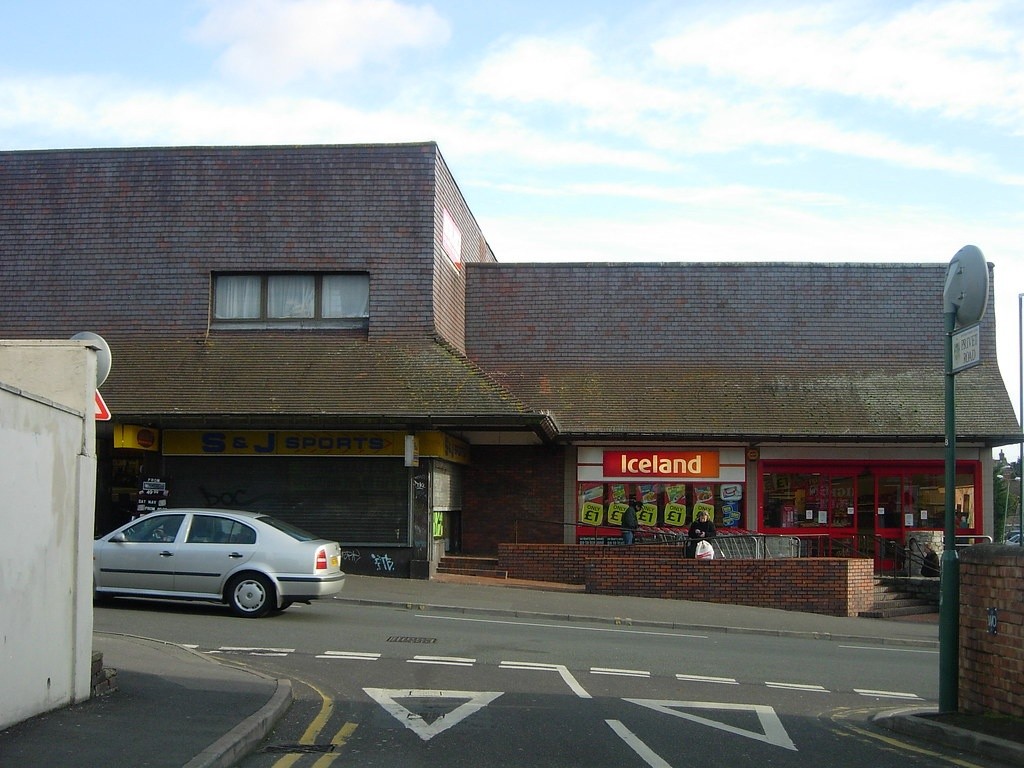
<box><xmin>695</xmin><ymin>540</ymin><xmax>713</xmax><ymax>560</ymax></box>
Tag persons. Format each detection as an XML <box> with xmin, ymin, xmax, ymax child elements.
<box><xmin>685</xmin><ymin>510</ymin><xmax>716</xmax><ymax>558</ymax></box>
<box><xmin>621</xmin><ymin>501</ymin><xmax>643</xmax><ymax>544</ymax></box>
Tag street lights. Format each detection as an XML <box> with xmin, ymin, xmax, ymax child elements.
<box><xmin>997</xmin><ymin>474</ymin><xmax>1020</xmax><ymax>544</ymax></box>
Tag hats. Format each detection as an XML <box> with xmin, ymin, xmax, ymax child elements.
<box><xmin>636</xmin><ymin>501</ymin><xmax>645</xmax><ymax>508</ymax></box>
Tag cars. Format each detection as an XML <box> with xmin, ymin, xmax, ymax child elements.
<box><xmin>93</xmin><ymin>508</ymin><xmax>346</xmax><ymax>618</ymax></box>
<box><xmin>1004</xmin><ymin>529</ymin><xmax>1020</xmax><ymax>546</ymax></box>
